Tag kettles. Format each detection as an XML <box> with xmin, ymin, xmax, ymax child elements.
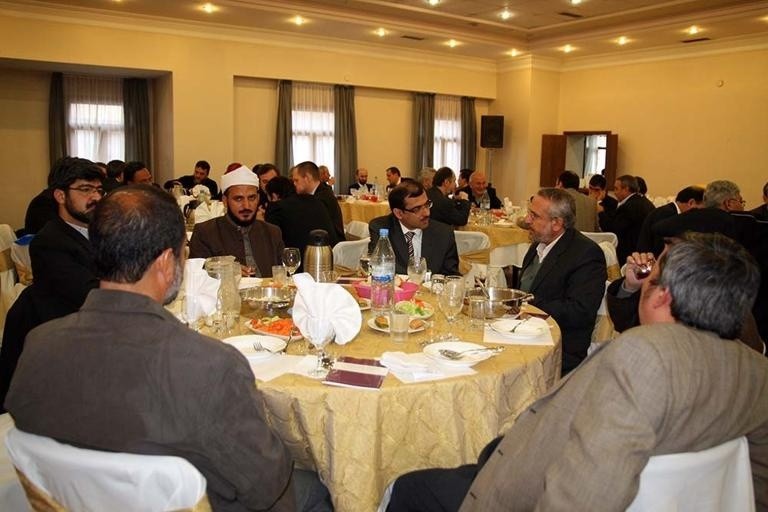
<box><xmin>303</xmin><ymin>230</ymin><xmax>334</xmax><ymax>283</ymax></box>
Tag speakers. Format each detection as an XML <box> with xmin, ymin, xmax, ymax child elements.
<box><xmin>480</xmin><ymin>115</ymin><xmax>504</xmax><ymax>148</ymax></box>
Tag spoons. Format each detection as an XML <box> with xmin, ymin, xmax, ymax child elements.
<box><xmin>423</xmin><ymin>341</ymin><xmax>491</xmax><ymax>367</ymax></box>
<box><xmin>438</xmin><ymin>345</ymin><xmax>505</xmax><ymax>361</ymax></box>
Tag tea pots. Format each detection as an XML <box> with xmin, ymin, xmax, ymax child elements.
<box><xmin>205</xmin><ymin>254</ymin><xmax>242</xmax><ymax>321</ymax></box>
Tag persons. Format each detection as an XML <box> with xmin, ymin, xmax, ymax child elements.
<box><xmin>28</xmin><ymin>157</ymin><xmax>107</xmax><ymax>297</ymax></box>
<box><xmin>554</xmin><ymin>172</ymin><xmax>600</xmax><ymax>231</ymax></box>
<box><xmin>586</xmin><ymin>175</ymin><xmax>616</xmax><ymax>228</ymax></box>
<box><xmin>424</xmin><ymin>167</ymin><xmax>470</xmax><ymax>226</ymax></box>
<box><xmin>164</xmin><ymin>160</ymin><xmax>217</xmax><ymax>199</ymax></box>
<box><xmin>417</xmin><ymin>168</ymin><xmax>437</xmax><ymax>190</ymax></box>
<box><xmin>257</xmin><ymin>162</ymin><xmax>280</xmax><ymax>220</ymax></box>
<box><xmin>292</xmin><ymin>162</ymin><xmax>346</xmax><ymax>242</ymax></box>
<box><xmin>4</xmin><ymin>184</ymin><xmax>333</xmax><ymax>512</ymax></box>
<box><xmin>456</xmin><ymin>169</ymin><xmax>472</xmax><ymax>190</ymax></box>
<box><xmin>188</xmin><ymin>165</ymin><xmax>289</xmax><ymax>279</ymax></box>
<box><xmin>598</xmin><ymin>174</ymin><xmax>650</xmax><ymax>256</ymax></box>
<box><xmin>347</xmin><ymin>168</ymin><xmax>376</xmax><ymax>195</ymax></box>
<box><xmin>25</xmin><ymin>186</ymin><xmax>57</xmax><ymax>233</ymax></box>
<box><xmin>701</xmin><ymin>180</ymin><xmax>749</xmax><ymax>216</ymax></box>
<box><xmin>457</xmin><ymin>171</ymin><xmax>503</xmax><ymax>209</ymax></box>
<box><xmin>747</xmin><ymin>181</ymin><xmax>768</xmax><ymax>221</ymax></box>
<box><xmin>636</xmin><ymin>184</ymin><xmax>706</xmax><ymax>254</ymax></box>
<box><xmin>104</xmin><ymin>160</ymin><xmax>124</xmax><ymax>194</ymax></box>
<box><xmin>633</xmin><ymin>176</ymin><xmax>656</xmax><ymax>212</ymax></box>
<box><xmin>264</xmin><ymin>176</ymin><xmax>338</xmax><ymax>248</ymax></box>
<box><xmin>607</xmin><ymin>232</ymin><xmax>767</xmax><ymax>355</ymax></box>
<box><xmin>318</xmin><ymin>166</ymin><xmax>335</xmax><ymax>186</ymax></box>
<box><xmin>124</xmin><ymin>161</ymin><xmax>154</xmax><ymax>188</ymax></box>
<box><xmin>385</xmin><ymin>167</ymin><xmax>415</xmax><ymax>193</ymax></box>
<box><xmin>384</xmin><ymin>231</ymin><xmax>768</xmax><ymax>512</ymax></box>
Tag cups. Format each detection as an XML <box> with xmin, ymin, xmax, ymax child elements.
<box><xmin>633</xmin><ymin>259</ymin><xmax>655</xmax><ymax>280</ymax></box>
<box><xmin>466</xmin><ymin>299</ymin><xmax>491</xmax><ymax>333</ymax></box>
<box><xmin>389</xmin><ymin>312</ymin><xmax>409</xmax><ymax>346</ymax></box>
<box><xmin>430</xmin><ymin>274</ymin><xmax>445</xmax><ymax>297</ymax></box>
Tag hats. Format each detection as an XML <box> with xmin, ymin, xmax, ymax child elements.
<box><xmin>220</xmin><ymin>165</ymin><xmax>259</xmax><ymax>195</ymax></box>
<box><xmin>224</xmin><ymin>163</ymin><xmax>241</xmax><ymax>174</ymax></box>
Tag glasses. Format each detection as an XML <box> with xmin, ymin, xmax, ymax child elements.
<box><xmin>729</xmin><ymin>200</ymin><xmax>746</xmax><ymax>206</ymax></box>
<box><xmin>402</xmin><ymin>199</ymin><xmax>435</xmax><ymax>214</ymax></box>
<box><xmin>68</xmin><ymin>184</ymin><xmax>104</xmax><ymax>194</ymax></box>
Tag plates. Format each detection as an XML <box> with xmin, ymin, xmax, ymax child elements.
<box><xmin>339</xmin><ymin>284</ymin><xmax>371</xmax><ymax>311</ymax></box>
<box><xmin>367</xmin><ymin>315</ymin><xmax>424</xmax><ymax>334</ymax></box>
<box><xmin>221</xmin><ymin>334</ymin><xmax>288</xmax><ymax>360</ymax></box>
<box><xmin>244</xmin><ymin>320</ymin><xmax>303</xmax><ymax>342</ymax></box>
<box><xmin>395</xmin><ymin>298</ymin><xmax>434</xmax><ymax>319</ymax></box>
<box><xmin>239</xmin><ymin>276</ymin><xmax>263</xmax><ymax>293</ymax></box>
<box><xmin>494</xmin><ymin>222</ymin><xmax>514</xmax><ymax>228</ymax></box>
<box><xmin>337</xmin><ymin>275</ymin><xmax>367</xmax><ymax>281</ymax></box>
<box><xmin>354</xmin><ymin>282</ymin><xmax>418</xmax><ymax>303</ymax></box>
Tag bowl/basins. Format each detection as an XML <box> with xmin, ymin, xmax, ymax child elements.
<box><xmin>240</xmin><ymin>286</ymin><xmax>296</xmax><ymax>319</ymax></box>
<box><xmin>461</xmin><ymin>287</ymin><xmax>534</xmax><ymax>319</ymax></box>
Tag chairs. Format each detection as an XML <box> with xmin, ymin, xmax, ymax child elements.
<box><xmin>344</xmin><ymin>220</ymin><xmax>369</xmax><ymax>241</ymax></box>
<box><xmin>11</xmin><ymin>232</ymin><xmax>33</xmax><ymax>285</ymax></box>
<box><xmin>332</xmin><ymin>236</ymin><xmax>370</xmax><ymax>278</ymax></box>
<box><xmin>624</xmin><ymin>436</ymin><xmax>760</xmax><ymax>512</ymax></box>
<box><xmin>581</xmin><ymin>231</ymin><xmax>619</xmax><ymax>269</ymax></box>
<box><xmin>646</xmin><ymin>192</ymin><xmax>677</xmax><ymax>207</ymax></box>
<box><xmin>0</xmin><ymin>223</ymin><xmax>28</xmax><ymax>316</ymax></box>
<box><xmin>597</xmin><ymin>242</ymin><xmax>622</xmax><ymax>281</ymax></box>
<box><xmin>455</xmin><ymin>230</ymin><xmax>492</xmax><ymax>257</ymax></box>
<box><xmin>0</xmin><ymin>410</ymin><xmax>212</xmax><ymax>512</ymax></box>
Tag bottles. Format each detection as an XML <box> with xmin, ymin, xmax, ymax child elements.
<box><xmin>372</xmin><ymin>176</ymin><xmax>379</xmax><ymax>195</ymax></box>
<box><xmin>480</xmin><ymin>189</ymin><xmax>490</xmax><ymax>215</ymax></box>
<box><xmin>369</xmin><ymin>229</ymin><xmax>396</xmax><ymax>313</ymax></box>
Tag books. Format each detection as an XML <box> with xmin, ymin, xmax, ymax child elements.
<box><xmin>515</xmin><ymin>311</ymin><xmax>551</xmax><ymax>323</ymax></box>
<box><xmin>335</xmin><ymin>274</ymin><xmax>369</xmax><ymax>287</ymax></box>
<box><xmin>321</xmin><ymin>356</ymin><xmax>386</xmax><ymax>392</ymax></box>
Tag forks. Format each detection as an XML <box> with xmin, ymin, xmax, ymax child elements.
<box><xmin>503</xmin><ymin>316</ymin><xmax>532</xmax><ymax>336</ymax></box>
<box><xmin>252</xmin><ymin>342</ymin><xmax>281</xmax><ymax>357</ymax></box>
<box><xmin>492</xmin><ymin>319</ymin><xmax>546</xmax><ymax>340</ymax></box>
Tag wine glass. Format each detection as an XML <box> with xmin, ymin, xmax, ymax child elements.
<box><xmin>182</xmin><ymin>296</ymin><xmax>205</xmax><ymax>334</ymax></box>
<box><xmin>272</xmin><ymin>266</ymin><xmax>287</xmax><ymax>286</ymax></box>
<box><xmin>444</xmin><ymin>275</ymin><xmax>465</xmax><ymax>295</ymax></box>
<box><xmin>359</xmin><ymin>253</ymin><xmax>372</xmax><ymax>281</ymax></box>
<box><xmin>437</xmin><ymin>292</ymin><xmax>463</xmax><ymax>341</ymax></box>
<box><xmin>282</xmin><ymin>248</ymin><xmax>301</xmax><ymax>284</ymax></box>
<box><xmin>407</xmin><ymin>256</ymin><xmax>427</xmax><ymax>285</ymax></box>
<box><xmin>322</xmin><ymin>271</ymin><xmax>337</xmax><ymax>283</ymax></box>
<box><xmin>307</xmin><ymin>318</ymin><xmax>335</xmax><ymax>379</ymax></box>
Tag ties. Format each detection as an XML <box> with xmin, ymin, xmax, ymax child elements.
<box><xmin>405</xmin><ymin>232</ymin><xmax>416</xmax><ymax>273</ymax></box>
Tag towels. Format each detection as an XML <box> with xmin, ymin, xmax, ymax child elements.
<box><xmin>185</xmin><ymin>258</ymin><xmax>220</xmax><ymax>321</ymax></box>
<box><xmin>379</xmin><ymin>351</ymin><xmax>444</xmax><ymax>382</ymax></box>
<box><xmin>292</xmin><ymin>272</ymin><xmax>361</xmax><ymax>346</ymax></box>
<box><xmin>194</xmin><ymin>201</ymin><xmax>224</xmax><ymax>223</ymax></box>
